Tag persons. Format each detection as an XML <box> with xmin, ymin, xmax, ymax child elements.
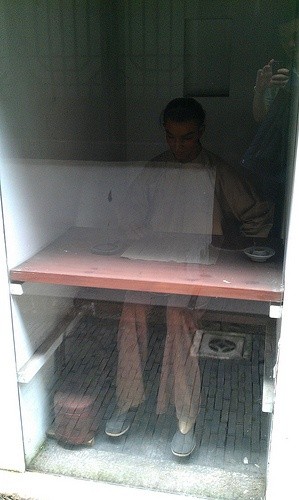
<box><xmin>253</xmin><ymin>9</ymin><xmax>299</xmax><ymax>123</ymax></box>
<box><xmin>105</xmin><ymin>98</ymin><xmax>263</xmax><ymax>457</ymax></box>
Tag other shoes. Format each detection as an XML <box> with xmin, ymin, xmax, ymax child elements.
<box><xmin>171</xmin><ymin>426</ymin><xmax>197</xmax><ymax>457</ymax></box>
<box><xmin>105</xmin><ymin>414</ymin><xmax>130</xmax><ymax>437</ymax></box>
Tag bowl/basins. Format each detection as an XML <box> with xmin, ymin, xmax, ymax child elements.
<box><xmin>243</xmin><ymin>245</ymin><xmax>275</xmax><ymax>262</ymax></box>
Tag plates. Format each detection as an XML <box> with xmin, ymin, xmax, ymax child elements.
<box><xmin>88</xmin><ymin>240</ymin><xmax>130</xmax><ymax>255</ymax></box>
<box><xmin>210</xmin><ymin>237</ymin><xmax>258</xmax><ymax>252</ymax></box>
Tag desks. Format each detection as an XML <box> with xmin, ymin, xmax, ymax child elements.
<box><xmin>9</xmin><ymin>226</ymin><xmax>285</xmax><ymax>320</ymax></box>
